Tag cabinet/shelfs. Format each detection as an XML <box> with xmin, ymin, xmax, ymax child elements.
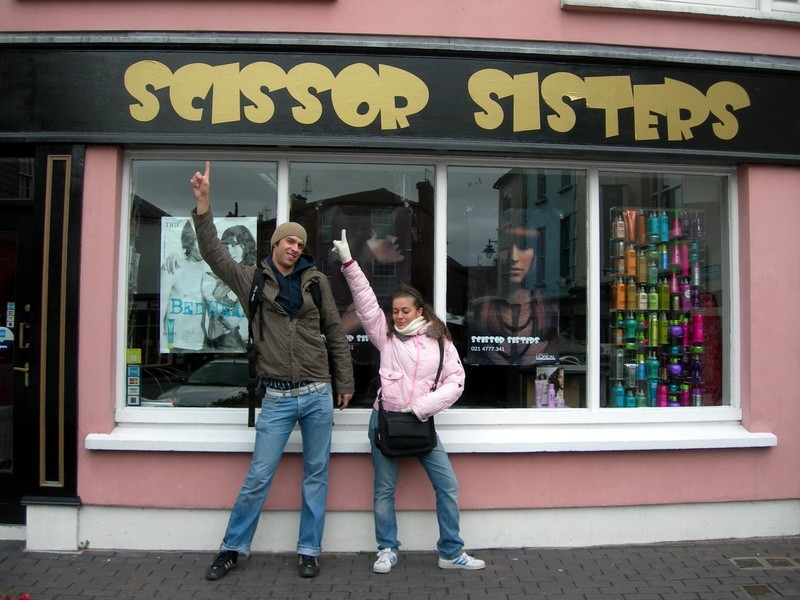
<box><xmin>610</xmin><ymin>206</ymin><xmax>703</xmax><ymax>408</ymax></box>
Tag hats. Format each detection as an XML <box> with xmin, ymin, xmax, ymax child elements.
<box><xmin>271</xmin><ymin>222</ymin><xmax>308</xmax><ymax>252</ymax></box>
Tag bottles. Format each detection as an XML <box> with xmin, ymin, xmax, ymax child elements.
<box><xmin>682</xmin><ymin>312</ymin><xmax>703</xmax><ymax>346</ymax></box>
<box><xmin>548</xmin><ymin>384</ymin><xmax>555</xmax><ymax>407</ymax></box>
<box><xmin>691</xmin><ymin>262</ymin><xmax>700</xmax><ymax>285</ymax></box>
<box><xmin>690</xmin><ymin>218</ymin><xmax>702</xmax><ymax>238</ymax></box>
<box><xmin>646</xmin><ymin>311</ymin><xmax>668</xmax><ymax>348</ymax></box>
<box><xmin>692</xmin><ymin>388</ymin><xmax>702</xmax><ymax>406</ymax></box>
<box><xmin>657</xmin><ymin>385</ymin><xmax>667</xmax><ymax>407</ymax></box>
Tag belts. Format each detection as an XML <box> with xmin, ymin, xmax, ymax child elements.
<box><xmin>266</xmin><ymin>382</ymin><xmax>327</xmax><ymax>397</ymax></box>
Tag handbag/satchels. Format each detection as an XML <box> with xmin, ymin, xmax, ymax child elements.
<box><xmin>374</xmin><ymin>410</ymin><xmax>437</xmax><ymax>458</ymax></box>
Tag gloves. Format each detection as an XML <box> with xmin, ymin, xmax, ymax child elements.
<box><xmin>331</xmin><ymin>229</ymin><xmax>353</xmax><ymax>264</ymax></box>
<box><xmin>401</xmin><ymin>407</ymin><xmax>412</xmax><ymax>412</ymax></box>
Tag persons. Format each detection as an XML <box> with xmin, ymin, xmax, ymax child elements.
<box><xmin>471</xmin><ymin>207</ymin><xmax>562</xmax><ymax>366</ymax></box>
<box><xmin>190</xmin><ymin>159</ymin><xmax>355</xmax><ymax>579</ymax></box>
<box><xmin>331</xmin><ymin>228</ymin><xmax>486</xmax><ymax>574</ymax></box>
<box><xmin>160</xmin><ymin>220</ymin><xmax>257</xmax><ymax>354</ymax></box>
<box><xmin>328</xmin><ymin>211</ymin><xmax>405</xmax><ymax>347</ymax></box>
<box><xmin>538</xmin><ymin>365</ymin><xmax>564</xmax><ymax>408</ymax></box>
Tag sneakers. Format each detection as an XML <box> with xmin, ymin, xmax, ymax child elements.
<box><xmin>438</xmin><ymin>552</ymin><xmax>486</xmax><ymax>570</ymax></box>
<box><xmin>206</xmin><ymin>550</ymin><xmax>239</xmax><ymax>580</ymax></box>
<box><xmin>298</xmin><ymin>554</ymin><xmax>321</xmax><ymax>577</ymax></box>
<box><xmin>373</xmin><ymin>547</ymin><xmax>399</xmax><ymax>573</ymax></box>
